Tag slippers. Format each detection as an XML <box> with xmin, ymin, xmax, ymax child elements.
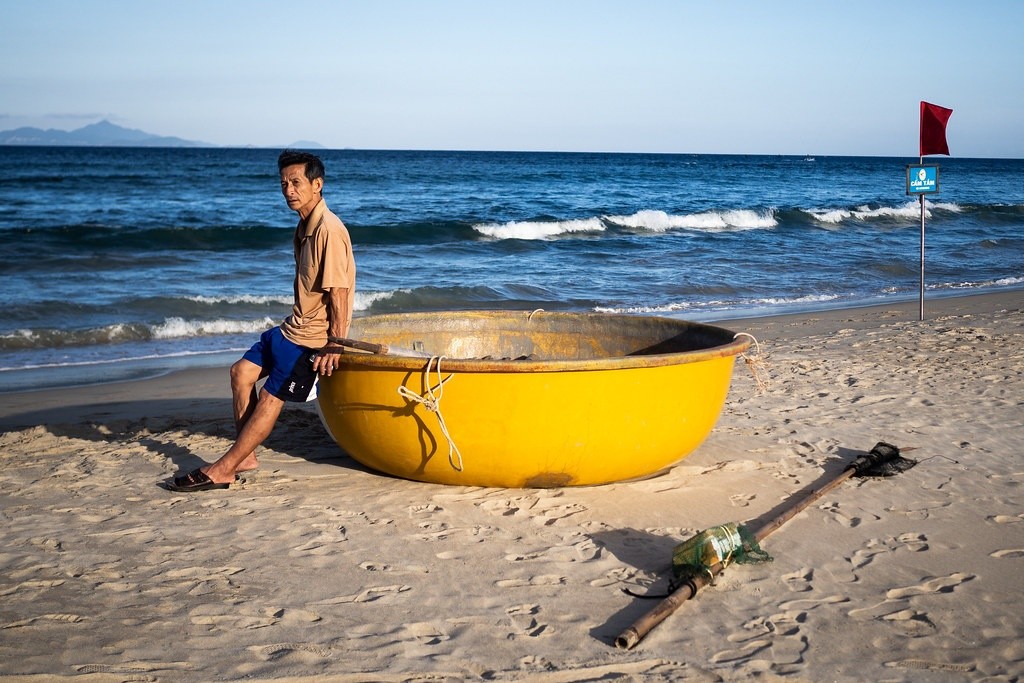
<box><xmin>166</xmin><ymin>468</ymin><xmax>230</xmax><ymax>492</ymax></box>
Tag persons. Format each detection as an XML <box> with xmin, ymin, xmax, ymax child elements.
<box><xmin>163</xmin><ymin>149</ymin><xmax>356</xmax><ymax>492</ymax></box>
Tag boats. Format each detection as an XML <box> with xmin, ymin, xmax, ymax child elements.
<box><xmin>309</xmin><ymin>306</ymin><xmax>753</xmax><ymax>489</ymax></box>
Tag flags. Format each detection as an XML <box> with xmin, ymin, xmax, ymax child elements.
<box><xmin>919</xmin><ymin>101</ymin><xmax>952</xmax><ymax>158</ymax></box>
